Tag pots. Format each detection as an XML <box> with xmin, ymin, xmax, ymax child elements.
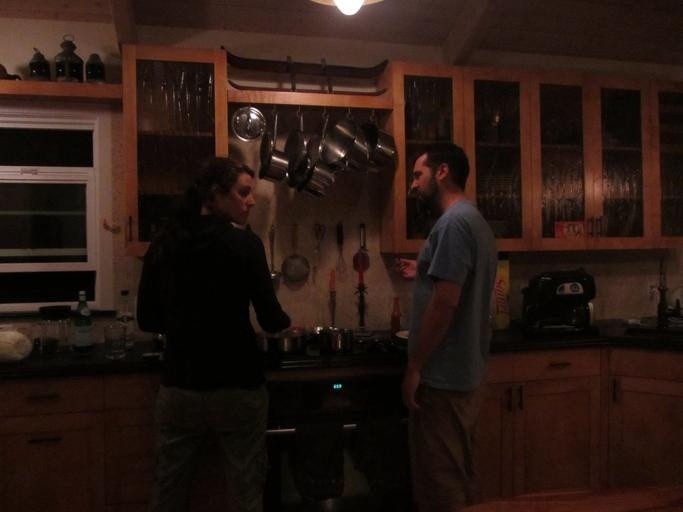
<box><xmin>231</xmin><ymin>102</ymin><xmax>397</xmax><ymax>202</ymax></box>
<box><xmin>255</xmin><ymin>332</ymin><xmax>295</xmax><ymax>362</ymax></box>
<box><xmin>318</xmin><ymin>326</ymin><xmax>355</xmax><ymax>356</ymax></box>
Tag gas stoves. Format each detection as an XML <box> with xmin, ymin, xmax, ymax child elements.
<box><xmin>252</xmin><ymin>332</ymin><xmax>411</xmax><ymax>369</ymax></box>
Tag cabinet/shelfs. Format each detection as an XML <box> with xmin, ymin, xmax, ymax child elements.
<box><xmin>0</xmin><ymin>377</ymin><xmax>228</xmax><ymax>512</ymax></box>
<box><xmin>651</xmin><ymin>62</ymin><xmax>682</xmax><ymax>251</ymax></box>
<box><xmin>531</xmin><ymin>67</ymin><xmax>651</xmax><ymax>253</ymax></box>
<box><xmin>394</xmin><ymin>64</ymin><xmax>533</xmax><ymax>255</ymax></box>
<box><xmin>482</xmin><ymin>347</ymin><xmax>683</xmax><ymax>497</ymax></box>
<box><xmin>121</xmin><ymin>43</ymin><xmax>229</xmax><ymax>257</ymax></box>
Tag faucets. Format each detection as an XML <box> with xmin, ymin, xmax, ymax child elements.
<box><xmin>656</xmin><ymin>285</ymin><xmax>681</xmax><ymax>325</ymax></box>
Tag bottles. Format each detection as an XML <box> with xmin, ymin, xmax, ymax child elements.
<box><xmin>38</xmin><ymin>304</ymin><xmax>72</xmax><ymax>360</ymax></box>
<box><xmin>117</xmin><ymin>289</ymin><xmax>136</xmax><ymax>349</ymax></box>
<box><xmin>73</xmin><ymin>289</ymin><xmax>94</xmax><ymax>353</ymax></box>
<box><xmin>390</xmin><ymin>296</ymin><xmax>403</xmax><ymax>336</ymax></box>
<box><xmin>55</xmin><ymin>41</ymin><xmax>84</xmax><ymax>83</ymax></box>
<box><xmin>29</xmin><ymin>53</ymin><xmax>51</xmax><ymax>80</ymax></box>
<box><xmin>85</xmin><ymin>53</ymin><xmax>106</xmax><ymax>83</ymax></box>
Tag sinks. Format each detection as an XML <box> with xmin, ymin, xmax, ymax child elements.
<box><xmin>624</xmin><ymin>325</ymin><xmax>683</xmax><ymax>349</ymax></box>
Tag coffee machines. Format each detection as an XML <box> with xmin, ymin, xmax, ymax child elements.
<box><xmin>520</xmin><ymin>268</ymin><xmax>596</xmax><ymax>338</ymax></box>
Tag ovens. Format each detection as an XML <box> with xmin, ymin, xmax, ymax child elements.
<box><xmin>262</xmin><ymin>376</ymin><xmax>418</xmax><ymax>512</ymax></box>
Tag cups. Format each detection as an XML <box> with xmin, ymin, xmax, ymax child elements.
<box><xmin>103</xmin><ymin>324</ymin><xmax>127</xmax><ymax>360</ymax></box>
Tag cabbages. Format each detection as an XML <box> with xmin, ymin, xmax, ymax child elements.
<box><xmin>1</xmin><ymin>330</ymin><xmax>32</xmax><ymax>360</ymax></box>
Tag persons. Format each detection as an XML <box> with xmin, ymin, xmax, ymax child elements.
<box><xmin>398</xmin><ymin>140</ymin><xmax>498</xmax><ymax>511</ymax></box>
<box><xmin>136</xmin><ymin>154</ymin><xmax>290</xmax><ymax>511</ymax></box>
